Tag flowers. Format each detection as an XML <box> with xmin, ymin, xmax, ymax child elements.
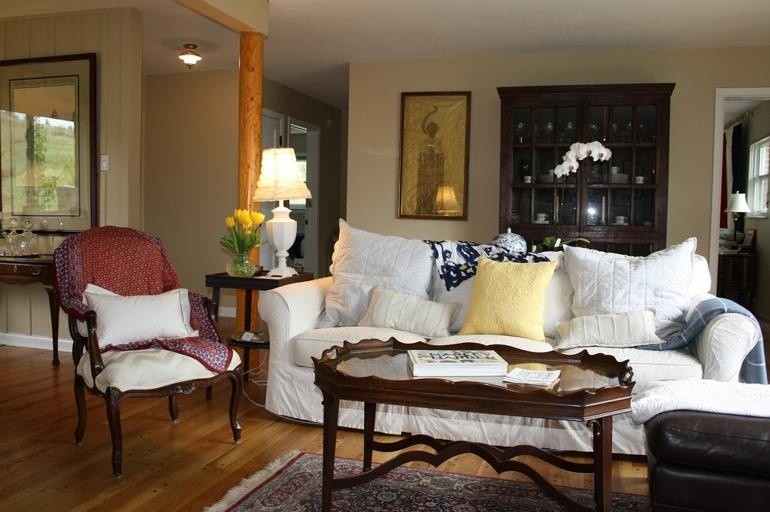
<box><xmin>533</xmin><ymin>142</ymin><xmax>612</xmax><ymax>255</ymax></box>
<box><xmin>220</xmin><ymin>208</ymin><xmax>265</xmax><ymax>275</ymax></box>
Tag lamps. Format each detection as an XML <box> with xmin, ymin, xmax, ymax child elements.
<box><xmin>252</xmin><ymin>147</ymin><xmax>312</xmax><ymax>278</ymax></box>
<box><xmin>724</xmin><ymin>190</ymin><xmax>752</xmax><ymax>249</ymax></box>
<box><xmin>176</xmin><ymin>43</ymin><xmax>203</xmax><ymax>72</ymax></box>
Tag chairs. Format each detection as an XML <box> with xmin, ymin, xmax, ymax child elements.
<box><xmin>51</xmin><ymin>225</ymin><xmax>243</xmax><ymax>479</ymax></box>
<box><xmin>631</xmin><ymin>378</ymin><xmax>770</xmax><ymax>512</ymax></box>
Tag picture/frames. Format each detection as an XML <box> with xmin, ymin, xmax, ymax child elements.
<box><xmin>0</xmin><ymin>51</ymin><xmax>98</xmax><ymax>240</ymax></box>
<box><xmin>397</xmin><ymin>90</ymin><xmax>472</xmax><ymax>221</ymax></box>
<box><xmin>740</xmin><ymin>228</ymin><xmax>756</xmax><ymax>250</ymax></box>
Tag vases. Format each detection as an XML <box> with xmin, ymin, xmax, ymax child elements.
<box><xmin>226</xmin><ymin>253</ymin><xmax>256</xmax><ymax>279</ymax></box>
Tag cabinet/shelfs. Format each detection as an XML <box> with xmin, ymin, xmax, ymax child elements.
<box><xmin>496</xmin><ymin>81</ymin><xmax>676</xmax><ymax>257</ymax></box>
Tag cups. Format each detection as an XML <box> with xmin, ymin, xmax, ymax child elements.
<box><xmin>636</xmin><ymin>176</ymin><xmax>649</xmax><ymax>184</ymax></box>
<box><xmin>615</xmin><ymin>215</ymin><xmax>628</xmax><ymax>225</ymax></box>
<box><xmin>524</xmin><ymin>176</ymin><xmax>537</xmax><ymax>184</ymax></box>
<box><xmin>612</xmin><ymin>166</ymin><xmax>622</xmax><ymax>175</ymax></box>
<box><xmin>549</xmin><ymin>169</ymin><xmax>554</xmax><ymax>175</ymax></box>
<box><xmin>536</xmin><ymin>213</ymin><xmax>549</xmax><ymax>222</ymax></box>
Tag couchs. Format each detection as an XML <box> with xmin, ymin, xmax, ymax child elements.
<box><xmin>257</xmin><ymin>253</ymin><xmax>760</xmax><ymax>457</ymax></box>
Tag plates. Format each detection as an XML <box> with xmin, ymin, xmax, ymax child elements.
<box><xmin>534</xmin><ymin>221</ymin><xmax>550</xmax><ymax>224</ymax></box>
<box><xmin>611</xmin><ymin>223</ymin><xmax>629</xmax><ymax>226</ymax></box>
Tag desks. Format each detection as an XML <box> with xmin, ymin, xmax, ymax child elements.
<box><xmin>0</xmin><ymin>253</ymin><xmax>60</xmax><ymax>367</ymax></box>
<box><xmin>716</xmin><ymin>251</ymin><xmax>758</xmax><ymax>311</ymax></box>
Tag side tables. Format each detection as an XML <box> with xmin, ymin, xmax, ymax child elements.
<box><xmin>204</xmin><ymin>270</ymin><xmax>314</xmax><ymax>396</ymax></box>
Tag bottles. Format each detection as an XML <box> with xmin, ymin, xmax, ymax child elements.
<box><xmin>16</xmin><ymin>217</ymin><xmax>40</xmax><ymax>256</ymax></box>
<box><xmin>3</xmin><ymin>218</ymin><xmax>22</xmax><ymax>257</ymax></box>
<box><xmin>0</xmin><ymin>211</ymin><xmax>10</xmax><ymax>257</ymax></box>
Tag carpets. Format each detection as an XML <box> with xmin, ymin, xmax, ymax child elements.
<box><xmin>201</xmin><ymin>448</ymin><xmax>652</xmax><ymax>512</ymax></box>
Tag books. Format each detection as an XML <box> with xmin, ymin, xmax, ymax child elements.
<box><xmin>502</xmin><ymin>367</ymin><xmax>561</xmax><ymax>387</ymax></box>
<box><xmin>407</xmin><ymin>349</ymin><xmax>508</xmax><ymax>377</ymax></box>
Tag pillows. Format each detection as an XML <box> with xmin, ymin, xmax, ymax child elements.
<box><xmin>83</xmin><ymin>284</ymin><xmax>201</xmax><ymax>349</ymax></box>
